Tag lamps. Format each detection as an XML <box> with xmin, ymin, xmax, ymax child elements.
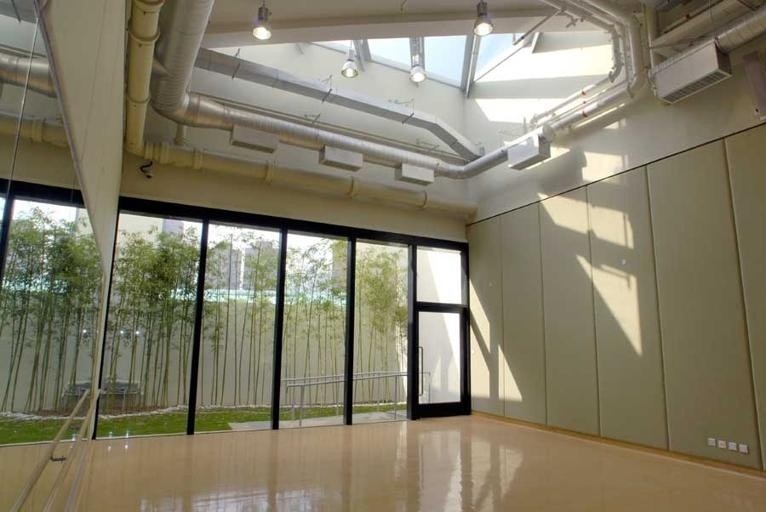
<box><xmin>472</xmin><ymin>0</ymin><xmax>495</xmax><ymax>37</ymax></box>
<box><xmin>408</xmin><ymin>37</ymin><xmax>426</xmax><ymax>83</ymax></box>
<box><xmin>341</xmin><ymin>40</ymin><xmax>359</xmax><ymax>78</ymax></box>
<box><xmin>252</xmin><ymin>0</ymin><xmax>272</xmax><ymax>40</ymax></box>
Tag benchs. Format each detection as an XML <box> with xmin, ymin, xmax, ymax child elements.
<box><xmin>61</xmin><ymin>382</ymin><xmax>139</xmax><ymax>412</ymax></box>
